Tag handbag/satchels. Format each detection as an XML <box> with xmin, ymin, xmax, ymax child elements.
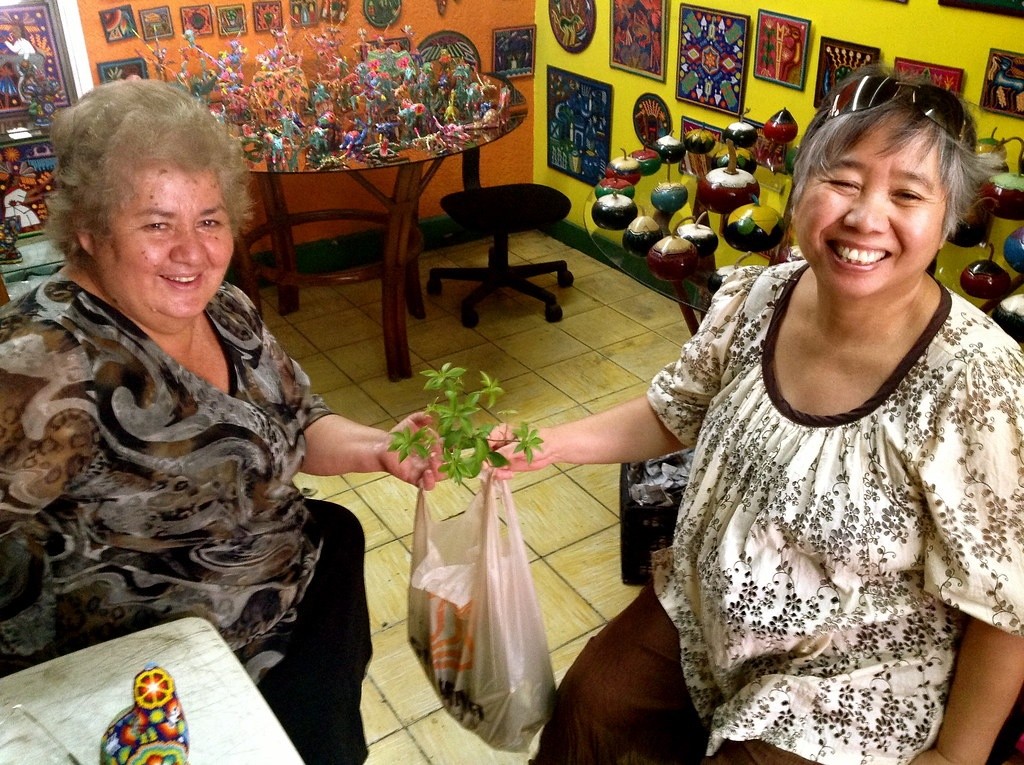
<box><xmin>407</xmin><ymin>467</ymin><xmax>556</xmax><ymax>751</ymax></box>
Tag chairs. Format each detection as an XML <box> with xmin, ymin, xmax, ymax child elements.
<box><xmin>425</xmin><ymin>145</ymin><xmax>573</xmax><ymax>328</ymax></box>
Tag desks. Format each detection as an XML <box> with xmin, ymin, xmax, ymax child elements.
<box><xmin>208</xmin><ymin>73</ymin><xmax>532</xmax><ymax>383</ymax></box>
<box><xmin>0</xmin><ymin>617</ymin><xmax>307</xmax><ymax>765</ymax></box>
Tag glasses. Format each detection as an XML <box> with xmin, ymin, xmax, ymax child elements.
<box><xmin>807</xmin><ymin>76</ymin><xmax>969</xmax><ymax>147</ymax></box>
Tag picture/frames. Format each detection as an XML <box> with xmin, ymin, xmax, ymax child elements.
<box><xmin>1</xmin><ymin>0</ymin><xmax>1023</xmax><ymax>188</ymax></box>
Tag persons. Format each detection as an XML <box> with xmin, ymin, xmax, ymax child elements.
<box><xmin>477</xmin><ymin>61</ymin><xmax>1023</xmax><ymax>764</ymax></box>
<box><xmin>0</xmin><ymin>79</ymin><xmax>457</xmax><ymax>765</ymax></box>
